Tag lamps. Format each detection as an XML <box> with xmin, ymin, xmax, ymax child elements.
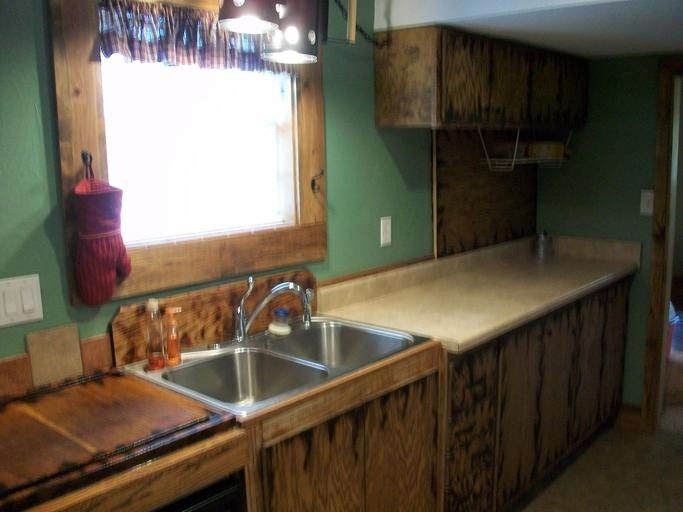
<box><xmin>218</xmin><ymin>1</ymin><xmax>319</xmax><ymax>66</ymax></box>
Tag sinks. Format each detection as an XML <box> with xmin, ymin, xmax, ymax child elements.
<box><xmin>162</xmin><ymin>347</ymin><xmax>328</xmax><ymax>418</ymax></box>
<box><xmin>265</xmin><ymin>317</ymin><xmax>431</xmax><ymax>366</ymax></box>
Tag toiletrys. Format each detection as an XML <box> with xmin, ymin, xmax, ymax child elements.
<box><xmin>143</xmin><ymin>298</ymin><xmax>166</xmax><ymax>370</ymax></box>
<box><xmin>165</xmin><ymin>307</ymin><xmax>183</xmax><ymax>367</ymax></box>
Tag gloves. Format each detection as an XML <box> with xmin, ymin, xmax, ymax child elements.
<box><xmin>71</xmin><ymin>176</ymin><xmax>131</xmax><ymax>309</ymax></box>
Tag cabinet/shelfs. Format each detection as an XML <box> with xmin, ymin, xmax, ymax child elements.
<box><xmin>244</xmin><ymin>276</ymin><xmax>629</xmax><ymax>512</ymax></box>
<box><xmin>374</xmin><ymin>25</ymin><xmax>588</xmax><ymax>130</ymax></box>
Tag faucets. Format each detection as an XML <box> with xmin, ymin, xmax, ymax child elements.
<box><xmin>245</xmin><ymin>281</ymin><xmax>312</xmax><ymax>336</ymax></box>
